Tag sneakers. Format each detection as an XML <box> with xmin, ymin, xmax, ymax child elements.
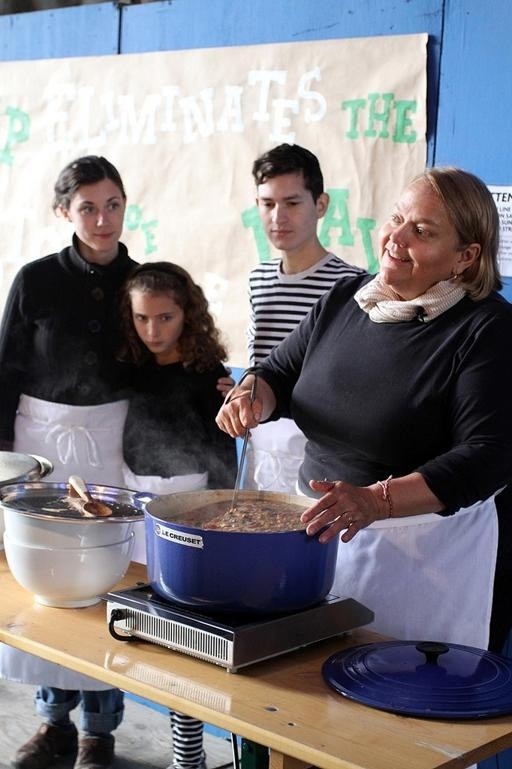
<box><xmin>73</xmin><ymin>730</ymin><xmax>116</xmax><ymax>769</ymax></box>
<box><xmin>12</xmin><ymin>720</ymin><xmax>76</xmax><ymax>769</ymax></box>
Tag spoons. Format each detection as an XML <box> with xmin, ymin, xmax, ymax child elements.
<box><xmin>67</xmin><ymin>474</ymin><xmax>112</xmax><ymax>517</ymax></box>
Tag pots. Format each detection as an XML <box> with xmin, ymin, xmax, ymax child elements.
<box><xmin>131</xmin><ymin>489</ymin><xmax>339</xmax><ymax>611</ymax></box>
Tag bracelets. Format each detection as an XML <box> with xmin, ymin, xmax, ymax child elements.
<box><xmin>377</xmin><ymin>474</ymin><xmax>395</xmax><ymax>518</ymax></box>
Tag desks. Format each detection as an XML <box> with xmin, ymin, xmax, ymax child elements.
<box><xmin>1</xmin><ymin>539</ymin><xmax>511</xmax><ymax>765</ymax></box>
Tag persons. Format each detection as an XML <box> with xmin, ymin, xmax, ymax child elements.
<box><xmin>112</xmin><ymin>260</ymin><xmax>239</xmax><ymax>769</ymax></box>
<box><xmin>2</xmin><ymin>153</ymin><xmax>236</xmax><ymax>767</ymax></box>
<box><xmin>239</xmin><ymin>142</ymin><xmax>367</xmax><ymax>490</ymax></box>
<box><xmin>215</xmin><ymin>169</ymin><xmax>510</xmax><ymax>663</ymax></box>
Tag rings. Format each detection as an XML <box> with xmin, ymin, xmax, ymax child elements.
<box><xmin>340</xmin><ymin>511</ymin><xmax>353</xmax><ymax>529</ymax></box>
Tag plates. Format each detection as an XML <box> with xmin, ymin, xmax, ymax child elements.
<box><xmin>0</xmin><ymin>449</ymin><xmax>54</xmax><ymax>496</ymax></box>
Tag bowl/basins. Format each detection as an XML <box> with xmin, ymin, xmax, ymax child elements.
<box><xmin>3</xmin><ymin>505</ymin><xmax>135</xmax><ymax>608</ymax></box>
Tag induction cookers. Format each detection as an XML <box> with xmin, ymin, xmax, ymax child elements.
<box><xmin>100</xmin><ymin>582</ymin><xmax>375</xmax><ymax>674</ymax></box>
